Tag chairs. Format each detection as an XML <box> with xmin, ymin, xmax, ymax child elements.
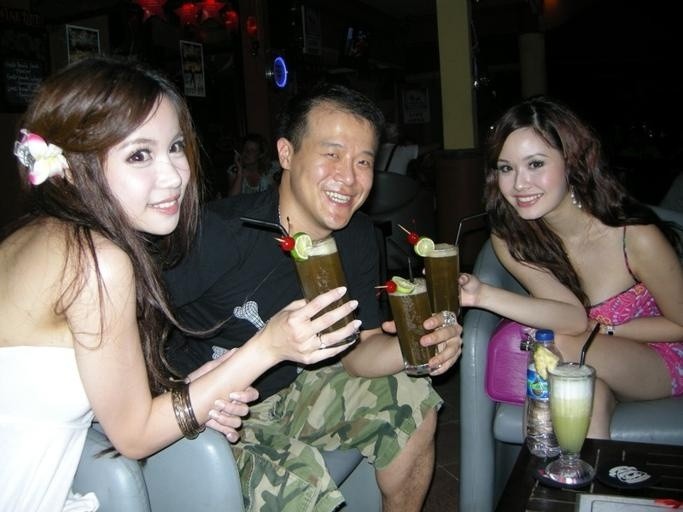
<box><xmin>444</xmin><ymin>209</ymin><xmax>495</xmax><ymax>276</ymax></box>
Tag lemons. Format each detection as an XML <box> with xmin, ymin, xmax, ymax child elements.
<box><xmin>291</xmin><ymin>231</ymin><xmax>313</xmax><ymax>262</ymax></box>
<box><xmin>392</xmin><ymin>275</ymin><xmax>414</xmax><ymax>294</ymax></box>
<box><xmin>414</xmin><ymin>235</ymin><xmax>435</xmax><ymax>257</ymax></box>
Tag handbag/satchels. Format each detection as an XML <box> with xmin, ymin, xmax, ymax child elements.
<box><xmin>485</xmin><ymin>318</ymin><xmax>536</xmax><ymax>406</ymax></box>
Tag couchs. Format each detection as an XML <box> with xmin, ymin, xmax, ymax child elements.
<box><xmin>366</xmin><ymin>172</ymin><xmax>435</xmax><ymax>271</ymax></box>
<box><xmin>137</xmin><ymin>424</ymin><xmax>388</xmax><ymax>511</ymax></box>
<box><xmin>71</xmin><ymin>426</ymin><xmax>150</xmax><ymax>510</ymax></box>
<box><xmin>456</xmin><ymin>198</ymin><xmax>682</xmax><ymax>511</ymax></box>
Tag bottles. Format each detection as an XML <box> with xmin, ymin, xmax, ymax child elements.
<box><xmin>524</xmin><ymin>328</ymin><xmax>561</xmax><ymax>460</ymax></box>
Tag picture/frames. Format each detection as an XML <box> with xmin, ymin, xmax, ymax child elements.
<box><xmin>65</xmin><ymin>23</ymin><xmax>101</xmax><ymax>66</ymax></box>
<box><xmin>401</xmin><ymin>87</ymin><xmax>431</xmax><ymax>124</ymax></box>
<box><xmin>180</xmin><ymin>40</ymin><xmax>206</xmax><ymax>97</ymax></box>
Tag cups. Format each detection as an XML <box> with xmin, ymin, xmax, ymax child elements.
<box><xmin>386</xmin><ymin>277</ymin><xmax>438</xmax><ymax>377</ymax></box>
<box><xmin>285</xmin><ymin>238</ymin><xmax>358</xmax><ymax>348</ymax></box>
<box><xmin>424</xmin><ymin>246</ymin><xmax>461</xmax><ymax>320</ymax></box>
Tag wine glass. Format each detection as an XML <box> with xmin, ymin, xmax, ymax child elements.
<box><xmin>546</xmin><ymin>363</ymin><xmax>596</xmax><ymax>487</ymax></box>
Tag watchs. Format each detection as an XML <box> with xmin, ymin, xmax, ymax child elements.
<box><xmin>606</xmin><ymin>325</ymin><xmax>615</xmax><ymax>337</ymax></box>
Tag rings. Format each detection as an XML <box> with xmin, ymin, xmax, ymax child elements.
<box><xmin>442</xmin><ymin>310</ymin><xmax>458</xmax><ymax>327</ymax></box>
<box><xmin>316</xmin><ymin>333</ymin><xmax>328</xmax><ymax>350</ymax></box>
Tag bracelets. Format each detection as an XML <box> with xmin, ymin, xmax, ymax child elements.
<box><xmin>172</xmin><ymin>382</ymin><xmax>208</xmax><ymax>440</ymax></box>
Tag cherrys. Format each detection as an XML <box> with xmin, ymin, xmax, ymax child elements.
<box><xmin>385</xmin><ymin>281</ymin><xmax>397</xmax><ymax>293</ymax></box>
<box><xmin>281</xmin><ymin>237</ymin><xmax>295</xmax><ymax>250</ymax></box>
<box><xmin>407</xmin><ymin>231</ymin><xmax>419</xmax><ymax>245</ymax></box>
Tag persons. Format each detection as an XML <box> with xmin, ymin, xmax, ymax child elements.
<box><xmin>420</xmin><ymin>93</ymin><xmax>682</xmax><ymax>441</ymax></box>
<box><xmin>0</xmin><ymin>56</ymin><xmax>361</xmax><ymax>511</ymax></box>
<box><xmin>133</xmin><ymin>77</ymin><xmax>463</xmax><ymax>512</ymax></box>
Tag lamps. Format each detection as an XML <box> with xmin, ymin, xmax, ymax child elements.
<box><xmin>265</xmin><ymin>54</ymin><xmax>288</xmax><ymax>88</ymax></box>
